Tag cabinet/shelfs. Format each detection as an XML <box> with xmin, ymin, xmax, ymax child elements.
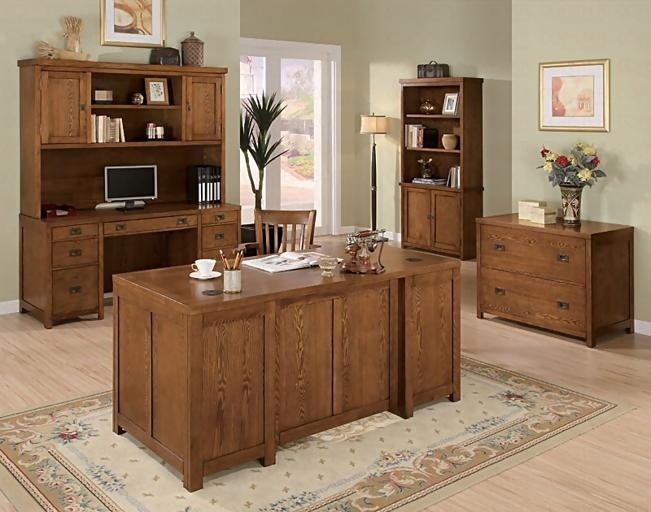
<box><xmin>401</xmin><ymin>187</ymin><xmax>482</xmax><ymax>261</ymax></box>
<box><xmin>17</xmin><ymin>213</ymin><xmax>103</xmax><ymax>329</ymax></box>
<box><xmin>399</xmin><ymin>76</ymin><xmax>484</xmax><ymax>192</ymax></box>
<box><xmin>17</xmin><ymin>58</ymin><xmax>229</xmax><ymax>220</ymax></box>
<box><xmin>162</xmin><ymin>206</ymin><xmax>242</xmax><ymax>266</ymax></box>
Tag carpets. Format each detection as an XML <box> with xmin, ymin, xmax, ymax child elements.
<box><xmin>0</xmin><ymin>353</ymin><xmax>639</xmax><ymax>512</ymax></box>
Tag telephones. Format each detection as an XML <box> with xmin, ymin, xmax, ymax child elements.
<box><xmin>341</xmin><ymin>230</ymin><xmax>388</xmax><ymax>273</ymax></box>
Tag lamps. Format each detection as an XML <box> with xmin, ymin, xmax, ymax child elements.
<box><xmin>359</xmin><ymin>111</ymin><xmax>389</xmax><ymax>242</ymax></box>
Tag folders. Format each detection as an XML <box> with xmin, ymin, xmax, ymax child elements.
<box><xmin>185</xmin><ymin>165</ymin><xmax>221</xmax><ymax>205</ymax></box>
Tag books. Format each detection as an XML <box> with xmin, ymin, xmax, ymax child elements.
<box><xmin>404</xmin><ymin>123</ymin><xmax>436</xmax><ymax>148</ymax></box>
<box><xmin>445</xmin><ymin>166</ymin><xmax>461</xmax><ymax>189</ymax></box>
<box><xmin>411</xmin><ymin>178</ymin><xmax>447</xmax><ymax>185</ymax></box>
<box><xmin>90</xmin><ymin>113</ymin><xmax>126</xmax><ymax>143</ymax></box>
<box><xmin>240</xmin><ymin>251</ymin><xmax>345</xmax><ymax>275</ymax></box>
<box><xmin>144</xmin><ymin>119</ymin><xmax>168</xmax><ymax>141</ymax></box>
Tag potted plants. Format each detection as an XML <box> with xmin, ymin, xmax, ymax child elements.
<box><xmin>535</xmin><ymin>140</ymin><xmax>606</xmax><ymax>185</ymax></box>
<box><xmin>239</xmin><ymin>88</ymin><xmax>289</xmax><ymax>255</ymax></box>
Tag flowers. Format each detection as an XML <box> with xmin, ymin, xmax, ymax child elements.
<box><xmin>417</xmin><ymin>157</ymin><xmax>432</xmax><ymax>166</ymax></box>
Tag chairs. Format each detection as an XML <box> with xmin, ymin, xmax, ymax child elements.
<box><xmin>254</xmin><ymin>210</ymin><xmax>317</xmax><ymax>255</ymax></box>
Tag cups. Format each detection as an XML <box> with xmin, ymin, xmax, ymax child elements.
<box><xmin>145</xmin><ymin>122</ymin><xmax>166</xmax><ymax>140</ymax></box>
<box><xmin>223</xmin><ymin>269</ymin><xmax>243</xmax><ymax>295</ymax></box>
<box><xmin>419</xmin><ymin>97</ymin><xmax>438</xmax><ymax>114</ymax></box>
<box><xmin>191</xmin><ymin>259</ymin><xmax>216</xmax><ymax>275</ymax></box>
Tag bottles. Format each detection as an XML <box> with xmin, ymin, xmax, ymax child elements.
<box><xmin>150</xmin><ymin>31</ymin><xmax>205</xmax><ymax>65</ymax></box>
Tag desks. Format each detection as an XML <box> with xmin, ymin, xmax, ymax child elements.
<box><xmin>111</xmin><ymin>243</ymin><xmax>461</xmax><ymax>495</ymax></box>
<box><xmin>475</xmin><ymin>213</ymin><xmax>635</xmax><ymax>348</ymax></box>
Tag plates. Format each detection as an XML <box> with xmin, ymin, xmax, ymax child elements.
<box><xmin>189</xmin><ymin>271</ymin><xmax>222</xmax><ymax>280</ymax></box>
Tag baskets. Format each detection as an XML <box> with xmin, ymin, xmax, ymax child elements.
<box><xmin>417</xmin><ymin>59</ymin><xmax>450</xmax><ymax>78</ymax></box>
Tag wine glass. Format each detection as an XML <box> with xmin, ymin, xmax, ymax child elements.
<box><xmin>318</xmin><ymin>256</ymin><xmax>338</xmax><ymax>277</ymax></box>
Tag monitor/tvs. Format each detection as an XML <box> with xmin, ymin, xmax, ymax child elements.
<box><xmin>104</xmin><ymin>164</ymin><xmax>159</xmax><ymax>212</ymax></box>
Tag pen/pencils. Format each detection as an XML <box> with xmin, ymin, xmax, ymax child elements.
<box><xmin>220</xmin><ymin>249</ymin><xmax>244</xmax><ymax>270</ymax></box>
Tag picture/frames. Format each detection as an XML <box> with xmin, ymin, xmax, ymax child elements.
<box><xmin>538</xmin><ymin>58</ymin><xmax>610</xmax><ymax>133</ymax></box>
<box><xmin>144</xmin><ymin>77</ymin><xmax>169</xmax><ymax>105</ymax></box>
<box><xmin>442</xmin><ymin>93</ymin><xmax>458</xmax><ymax>115</ymax></box>
<box><xmin>100</xmin><ymin>0</ymin><xmax>166</xmax><ymax>47</ymax></box>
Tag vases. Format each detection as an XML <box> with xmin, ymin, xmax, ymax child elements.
<box><xmin>419</xmin><ymin>165</ymin><xmax>432</xmax><ymax>178</ymax></box>
<box><xmin>559</xmin><ymin>182</ymin><xmax>584</xmax><ymax>227</ymax></box>
